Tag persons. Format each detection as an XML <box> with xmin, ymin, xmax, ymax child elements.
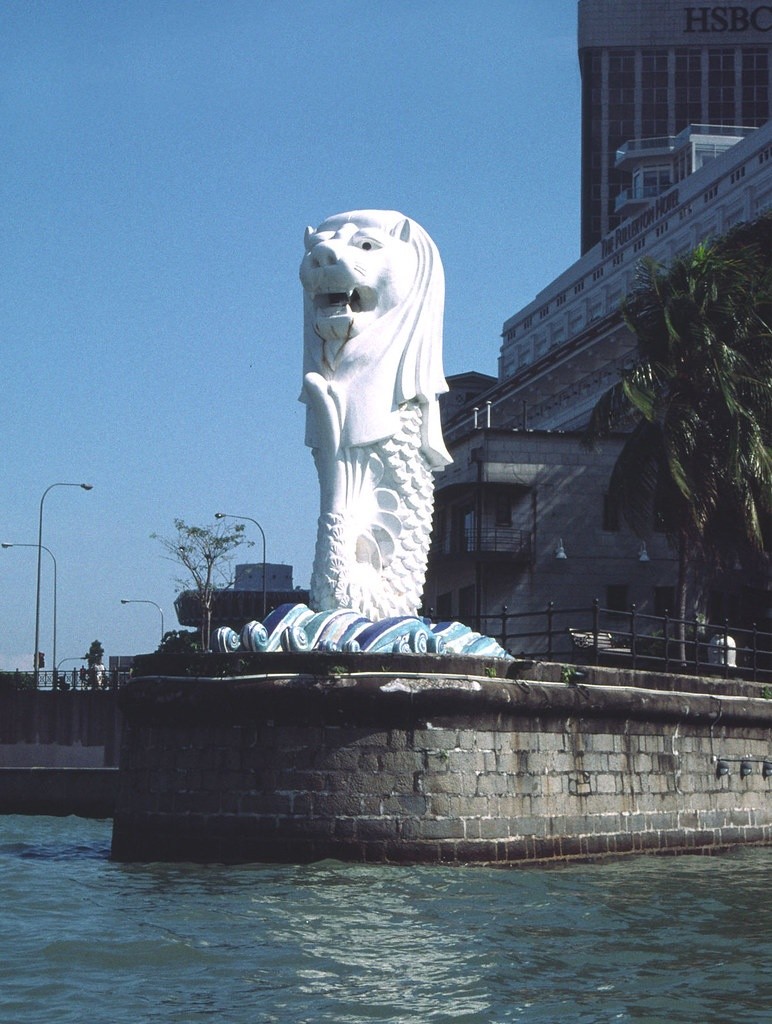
<box><xmin>71</xmin><ymin>667</ymin><xmax>78</xmax><ymax>686</ymax></box>
<box><xmin>80</xmin><ymin>665</ymin><xmax>86</xmax><ymax>684</ymax></box>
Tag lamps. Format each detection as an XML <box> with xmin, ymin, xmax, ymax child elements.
<box><xmin>555</xmin><ymin>538</ymin><xmax>568</xmax><ymax>559</ymax></box>
<box><xmin>638</xmin><ymin>540</ymin><xmax>650</xmax><ymax>561</ymax></box>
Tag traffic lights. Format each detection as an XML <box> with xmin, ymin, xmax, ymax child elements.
<box><xmin>39</xmin><ymin>652</ymin><xmax>47</xmax><ymax>669</ymax></box>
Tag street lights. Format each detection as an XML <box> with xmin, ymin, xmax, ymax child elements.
<box><xmin>32</xmin><ymin>483</ymin><xmax>94</xmax><ymax>686</ymax></box>
<box><xmin>215</xmin><ymin>512</ymin><xmax>267</xmax><ymax>620</ymax></box>
<box><xmin>1</xmin><ymin>542</ymin><xmax>57</xmax><ymax>688</ymax></box>
<box><xmin>121</xmin><ymin>599</ymin><xmax>163</xmax><ymax>647</ymax></box>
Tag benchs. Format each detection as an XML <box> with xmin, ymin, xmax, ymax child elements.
<box><xmin>568</xmin><ymin>628</ymin><xmax>633</xmax><ymax>667</ymax></box>
